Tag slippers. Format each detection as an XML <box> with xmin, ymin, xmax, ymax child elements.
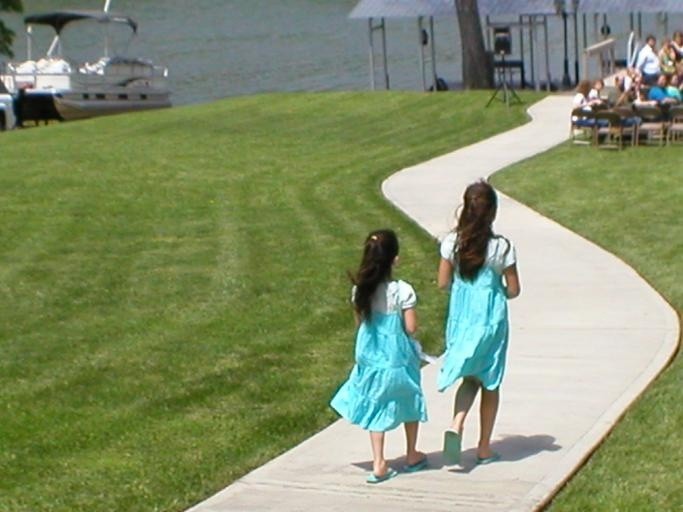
<box><xmin>402</xmin><ymin>455</ymin><xmax>430</xmax><ymax>473</ymax></box>
<box><xmin>365</xmin><ymin>466</ymin><xmax>398</xmax><ymax>484</ymax></box>
<box><xmin>442</xmin><ymin>428</ymin><xmax>463</xmax><ymax>468</ymax></box>
<box><xmin>475</xmin><ymin>451</ymin><xmax>502</xmax><ymax>465</ymax></box>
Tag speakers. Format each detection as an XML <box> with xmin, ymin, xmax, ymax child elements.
<box><xmin>493</xmin><ymin>26</ymin><xmax>511</xmax><ymax>54</ymax></box>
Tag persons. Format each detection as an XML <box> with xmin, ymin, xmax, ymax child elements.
<box><xmin>330</xmin><ymin>229</ymin><xmax>430</xmax><ymax>482</ymax></box>
<box><xmin>437</xmin><ymin>180</ymin><xmax>520</xmax><ymax>464</ymax></box>
<box><xmin>571</xmin><ymin>30</ymin><xmax>683</xmax><ymax>137</ymax></box>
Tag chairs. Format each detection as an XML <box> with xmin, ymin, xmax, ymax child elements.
<box><xmin>570</xmin><ymin>68</ymin><xmax>682</xmax><ymax>151</ymax></box>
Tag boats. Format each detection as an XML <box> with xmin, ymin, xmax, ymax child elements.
<box><xmin>0</xmin><ymin>0</ymin><xmax>176</xmax><ymax>126</ymax></box>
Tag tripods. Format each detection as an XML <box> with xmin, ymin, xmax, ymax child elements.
<box><xmin>486</xmin><ymin>58</ymin><xmax>523</xmax><ymax>110</ymax></box>
